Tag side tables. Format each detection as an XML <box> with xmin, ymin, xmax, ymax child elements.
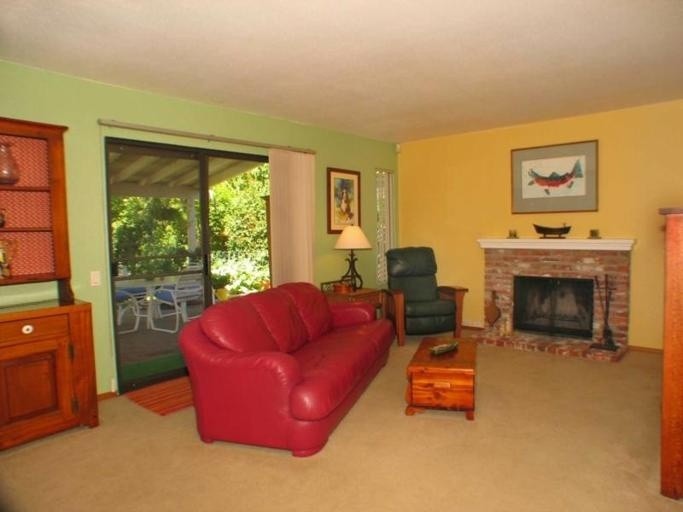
<box><xmin>327</xmin><ymin>286</ymin><xmax>387</xmax><ymax>318</ymax></box>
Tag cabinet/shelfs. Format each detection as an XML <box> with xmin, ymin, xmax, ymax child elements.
<box><xmin>0</xmin><ymin>115</ymin><xmax>75</xmax><ymax>302</ymax></box>
<box><xmin>0</xmin><ymin>299</ymin><xmax>98</xmax><ymax>450</ymax></box>
<box><xmin>658</xmin><ymin>206</ymin><xmax>683</xmax><ymax>500</ymax></box>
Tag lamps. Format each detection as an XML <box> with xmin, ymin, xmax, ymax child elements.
<box><xmin>333</xmin><ymin>225</ymin><xmax>372</xmax><ymax>292</ymax></box>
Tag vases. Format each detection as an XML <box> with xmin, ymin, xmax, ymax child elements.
<box><xmin>0</xmin><ymin>141</ymin><xmax>20</xmax><ymax>185</ymax></box>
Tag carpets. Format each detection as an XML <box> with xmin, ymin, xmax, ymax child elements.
<box><xmin>125</xmin><ymin>373</ymin><xmax>195</xmax><ymax>415</ymax></box>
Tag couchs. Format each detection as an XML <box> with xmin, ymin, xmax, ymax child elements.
<box><xmin>178</xmin><ymin>282</ymin><xmax>394</xmax><ymax>457</ymax></box>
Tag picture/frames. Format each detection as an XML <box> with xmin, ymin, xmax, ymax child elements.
<box><xmin>327</xmin><ymin>167</ymin><xmax>361</xmax><ymax>234</ymax></box>
<box><xmin>510</xmin><ymin>139</ymin><xmax>598</xmax><ymax>213</ymax></box>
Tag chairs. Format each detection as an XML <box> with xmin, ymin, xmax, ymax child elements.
<box><xmin>115</xmin><ymin>279</ymin><xmax>205</xmax><ymax>333</ymax></box>
<box><xmin>384</xmin><ymin>246</ymin><xmax>468</xmax><ymax>345</ymax></box>
